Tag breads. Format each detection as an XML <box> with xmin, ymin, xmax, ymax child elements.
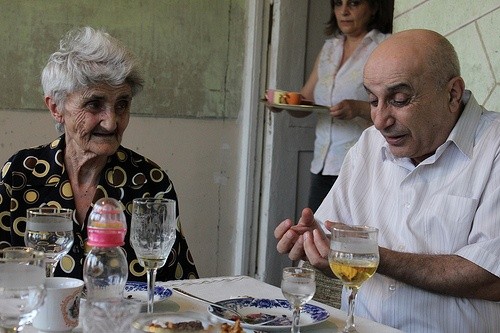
<box><xmin>148</xmin><ymin>321</ymin><xmax>205</xmax><ymax>333</ymax></box>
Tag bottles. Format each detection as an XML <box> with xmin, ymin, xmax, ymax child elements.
<box><xmin>82</xmin><ymin>198</ymin><xmax>128</xmax><ymax>298</ymax></box>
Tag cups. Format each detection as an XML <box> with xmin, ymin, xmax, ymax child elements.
<box><xmin>0</xmin><ymin>247</ymin><xmax>45</xmax><ymax>333</ymax></box>
<box><xmin>266</xmin><ymin>89</ymin><xmax>303</xmax><ymax>105</ymax></box>
<box><xmin>31</xmin><ymin>277</ymin><xmax>84</xmax><ymax>333</ymax></box>
<box><xmin>78</xmin><ymin>297</ymin><xmax>141</xmax><ymax>333</ymax></box>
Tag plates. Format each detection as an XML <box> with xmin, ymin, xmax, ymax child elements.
<box><xmin>207</xmin><ymin>296</ymin><xmax>330</xmax><ymax>333</ymax></box>
<box><xmin>82</xmin><ymin>280</ymin><xmax>173</xmax><ymax>308</ymax></box>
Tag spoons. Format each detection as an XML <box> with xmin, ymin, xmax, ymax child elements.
<box><xmin>173</xmin><ymin>287</ymin><xmax>280</xmax><ymax>326</ymax></box>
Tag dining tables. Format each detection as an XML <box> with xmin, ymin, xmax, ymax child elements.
<box><xmin>0</xmin><ymin>276</ymin><xmax>405</xmax><ymax>333</ymax></box>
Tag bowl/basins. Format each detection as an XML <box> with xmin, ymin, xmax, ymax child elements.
<box><xmin>129</xmin><ymin>311</ymin><xmax>222</xmax><ymax>333</ymax></box>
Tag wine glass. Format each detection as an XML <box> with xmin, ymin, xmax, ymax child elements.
<box><xmin>129</xmin><ymin>197</ymin><xmax>177</xmax><ymax>312</ymax></box>
<box><xmin>26</xmin><ymin>207</ymin><xmax>75</xmax><ymax>277</ymax></box>
<box><xmin>281</xmin><ymin>266</ymin><xmax>316</xmax><ymax>333</ymax></box>
<box><xmin>328</xmin><ymin>224</ymin><xmax>380</xmax><ymax>333</ymax></box>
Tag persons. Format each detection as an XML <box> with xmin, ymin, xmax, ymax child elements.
<box><xmin>274</xmin><ymin>29</ymin><xmax>500</xmax><ymax>333</ymax></box>
<box><xmin>0</xmin><ymin>27</ymin><xmax>199</xmax><ymax>283</ymax></box>
<box><xmin>268</xmin><ymin>0</ymin><xmax>391</xmax><ymax>214</ymax></box>
